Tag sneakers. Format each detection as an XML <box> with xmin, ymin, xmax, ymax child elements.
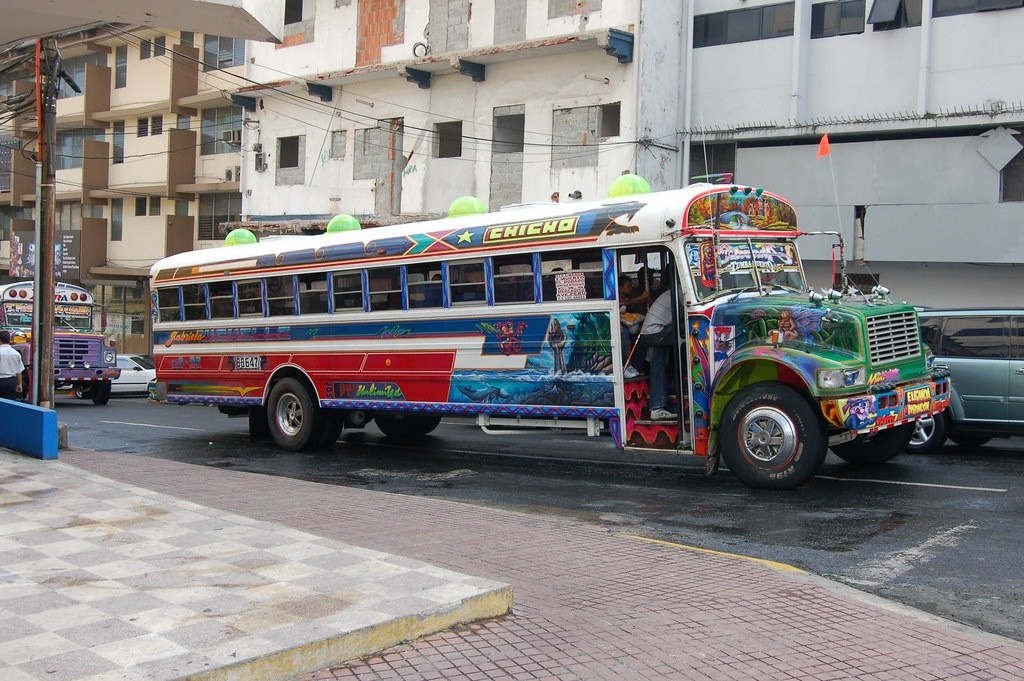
<box><xmin>651</xmin><ymin>407</ymin><xmax>678</xmax><ymax>421</ymax></box>
<box><xmin>624</xmin><ymin>365</ymin><xmax>639</xmax><ymax>378</ymax></box>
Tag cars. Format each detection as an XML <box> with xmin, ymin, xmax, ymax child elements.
<box><xmin>74</xmin><ymin>353</ymin><xmax>156</xmax><ymax>400</ymax></box>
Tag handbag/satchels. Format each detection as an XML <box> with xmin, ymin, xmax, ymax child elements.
<box><xmin>619</xmin><ymin>312</ymin><xmax>646</xmax><ymax>327</ymax></box>
<box><xmin>628</xmin><ymin>323</ymin><xmax>640</xmax><ymax>335</ymax></box>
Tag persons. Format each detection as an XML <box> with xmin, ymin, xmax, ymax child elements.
<box><xmin>0</xmin><ymin>331</ymin><xmax>25</xmax><ymax>401</ymax></box>
<box><xmin>618</xmin><ymin>263</ymin><xmax>678</xmax><ymax>420</ymax></box>
<box><xmin>423</xmin><ymin>268</ymin><xmax>566</xmax><ymax>307</ymax></box>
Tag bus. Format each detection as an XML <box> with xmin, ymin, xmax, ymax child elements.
<box><xmin>1</xmin><ymin>281</ymin><xmax>121</xmax><ymax>407</ymax></box>
<box><xmin>144</xmin><ymin>172</ymin><xmax>956</xmax><ymax>493</ymax></box>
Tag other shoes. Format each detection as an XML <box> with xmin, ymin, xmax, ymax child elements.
<box><xmin>639</xmin><ymin>365</ymin><xmax>650</xmax><ymax>376</ymax></box>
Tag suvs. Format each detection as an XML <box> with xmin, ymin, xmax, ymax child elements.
<box><xmin>904</xmin><ymin>303</ymin><xmax>1024</xmax><ymax>453</ymax></box>
